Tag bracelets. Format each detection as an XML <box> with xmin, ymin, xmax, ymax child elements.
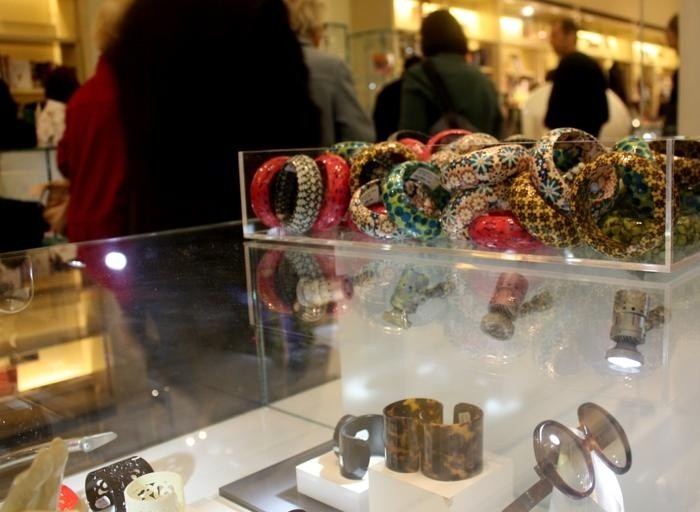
<box><xmin>384</xmin><ymin>390</ymin><xmax>486</xmax><ymax>481</ymax></box>
<box><xmin>248</xmin><ymin>119</ymin><xmax>699</xmax><ymax>265</ymax></box>
<box><xmin>123</xmin><ymin>471</ymin><xmax>187</xmax><ymax>511</ymax></box>
<box><xmin>85</xmin><ymin>454</ymin><xmax>154</xmax><ymax>511</ymax></box>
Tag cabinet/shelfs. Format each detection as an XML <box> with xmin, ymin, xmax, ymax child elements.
<box><xmin>402</xmin><ymin>1</ymin><xmax>676</xmax><ymax>126</ymax></box>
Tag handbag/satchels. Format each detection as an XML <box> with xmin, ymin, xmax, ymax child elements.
<box><xmin>416</xmin><ymin>55</ymin><xmax>480</xmax><ymax>138</ymax></box>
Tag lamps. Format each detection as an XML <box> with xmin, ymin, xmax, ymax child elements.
<box><xmin>295</xmin><ymin>259</ymin><xmax>671</xmax><ymax>371</ymax></box>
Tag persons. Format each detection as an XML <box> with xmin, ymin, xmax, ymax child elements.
<box><xmin>286</xmin><ymin>0</ymin><xmax>373</xmax><ymax>143</ymax></box>
<box><xmin>58</xmin><ymin>0</ymin><xmax>326</xmax><ymax>246</ymax></box>
<box><xmin>0</xmin><ymin>78</ymin><xmax>43</xmax><ymax>248</ymax></box>
<box><xmin>657</xmin><ymin>9</ymin><xmax>680</xmax><ymax>135</ymax></box>
<box><xmin>78</xmin><ymin>241</ymin><xmax>331</xmax><ymax>445</ymax></box>
<box><xmin>34</xmin><ymin>64</ymin><xmax>81</xmax><ymax>149</ymax></box>
<box><xmin>373</xmin><ymin>8</ymin><xmax>633</xmax><ymax>145</ymax></box>
